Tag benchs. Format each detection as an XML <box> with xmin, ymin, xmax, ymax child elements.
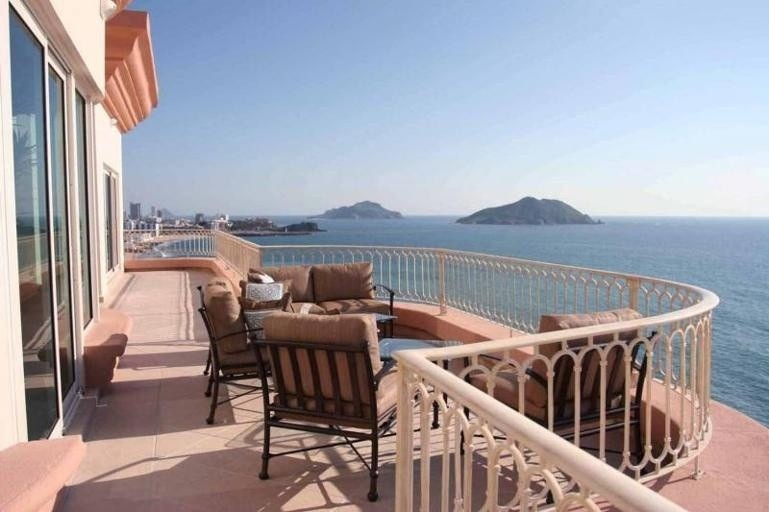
<box><xmin>238</xmin><ymin>261</ymin><xmax>396</xmax><ymax>343</ymax></box>
<box><xmin>198</xmin><ymin>277</ymin><xmax>275</xmax><ymax>424</ymax></box>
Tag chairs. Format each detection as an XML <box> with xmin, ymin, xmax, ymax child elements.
<box><xmin>257</xmin><ymin>312</ymin><xmax>418</xmax><ymax>502</ymax></box>
<box><xmin>461</xmin><ymin>307</ymin><xmax>658</xmax><ymax>503</ymax></box>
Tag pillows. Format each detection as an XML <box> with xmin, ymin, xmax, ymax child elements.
<box><xmin>239</xmin><ymin>268</ymin><xmax>296</xmax><ymax>342</ymax></box>
